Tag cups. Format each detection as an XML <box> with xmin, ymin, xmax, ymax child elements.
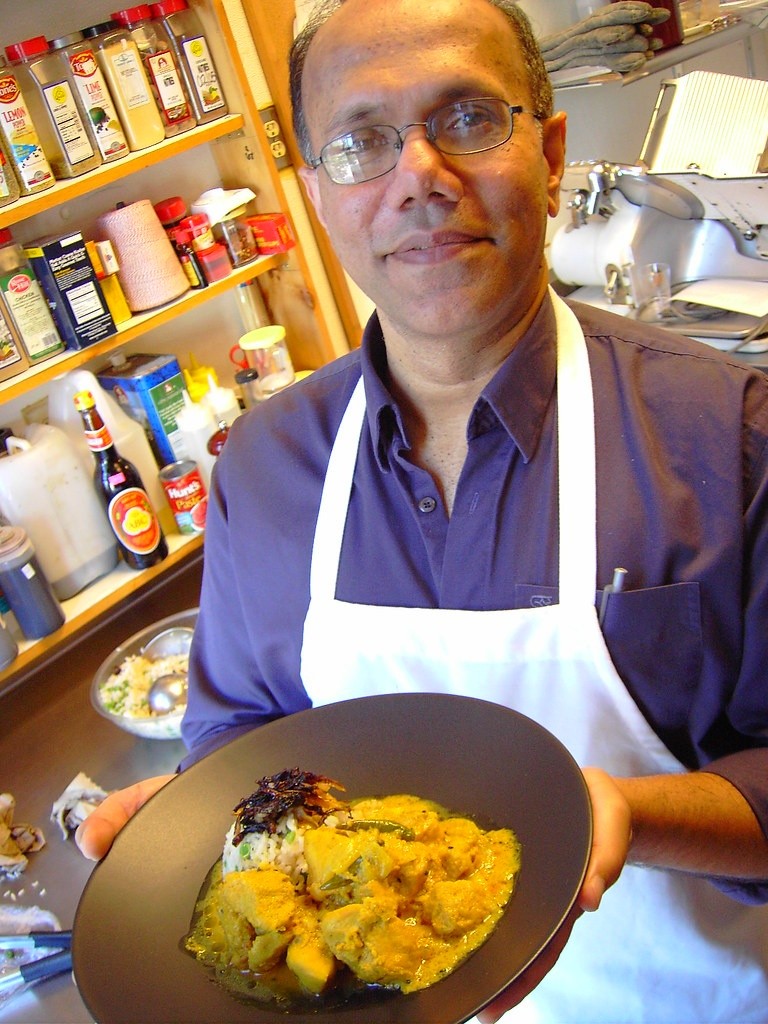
<box><xmin>642</xmin><ymin>263</ymin><xmax>671</xmax><ymax>302</ymax></box>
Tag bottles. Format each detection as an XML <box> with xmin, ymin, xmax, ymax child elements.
<box><xmin>0</xmin><ymin>526</ymin><xmax>66</xmax><ymax>638</ymax></box>
<box><xmin>73</xmin><ymin>391</ymin><xmax>169</xmax><ymax>570</ymax></box>
<box><xmin>176</xmin><ymin>353</ymin><xmax>263</xmax><ymax>498</ymax></box>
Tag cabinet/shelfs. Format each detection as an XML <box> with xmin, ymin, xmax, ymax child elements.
<box><xmin>0</xmin><ymin>0</ymin><xmax>351</xmax><ymax>691</ymax></box>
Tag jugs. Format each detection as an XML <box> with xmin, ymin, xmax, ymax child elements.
<box><xmin>0</xmin><ymin>368</ymin><xmax>178</xmax><ymax>601</ymax></box>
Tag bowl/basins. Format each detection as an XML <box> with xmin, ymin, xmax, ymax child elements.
<box><xmin>90</xmin><ymin>606</ymin><xmax>204</xmax><ymax>740</ymax></box>
<box><xmin>141</xmin><ymin>628</ymin><xmax>194</xmax><ymax>660</ymax></box>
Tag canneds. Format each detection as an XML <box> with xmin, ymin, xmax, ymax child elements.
<box><xmin>153</xmin><ymin>197</ymin><xmax>209</xmax><ymax>290</ymax></box>
<box><xmin>0</xmin><ymin>227</ymin><xmax>68</xmax><ymax>386</ymax></box>
<box><xmin>0</xmin><ymin>0</ymin><xmax>232</xmax><ymax>207</ymax></box>
<box><xmin>159</xmin><ymin>460</ymin><xmax>210</xmax><ymax>537</ymax></box>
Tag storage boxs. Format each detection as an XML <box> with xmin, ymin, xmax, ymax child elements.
<box><xmin>249</xmin><ymin>214</ymin><xmax>296</xmax><ymax>255</ymax></box>
<box><xmin>24</xmin><ymin>232</ymin><xmax>119</xmax><ymax>351</ymax></box>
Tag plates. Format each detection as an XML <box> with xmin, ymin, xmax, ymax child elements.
<box><xmin>69</xmin><ymin>691</ymin><xmax>592</xmax><ymax>1024</ymax></box>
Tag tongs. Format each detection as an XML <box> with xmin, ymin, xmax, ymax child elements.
<box><xmin>0</xmin><ymin>929</ymin><xmax>71</xmax><ymax>995</ymax></box>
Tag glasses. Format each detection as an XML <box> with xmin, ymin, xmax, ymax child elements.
<box><xmin>307</xmin><ymin>96</ymin><xmax>547</xmax><ymax>186</ymax></box>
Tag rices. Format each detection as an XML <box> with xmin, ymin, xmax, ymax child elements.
<box><xmin>222</xmin><ymin>808</ymin><xmax>336</xmax><ymax>874</ymax></box>
<box><xmin>97</xmin><ymin>645</ymin><xmax>188</xmax><ymax>717</ymax></box>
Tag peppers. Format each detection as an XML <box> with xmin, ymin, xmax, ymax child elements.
<box><xmin>335</xmin><ymin>820</ymin><xmax>414</xmax><ymax>839</ymax></box>
<box><xmin>320</xmin><ymin>853</ymin><xmax>365</xmax><ymax>890</ymax></box>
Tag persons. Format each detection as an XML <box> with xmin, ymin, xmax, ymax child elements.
<box><xmin>74</xmin><ymin>0</ymin><xmax>768</xmax><ymax>1024</ymax></box>
